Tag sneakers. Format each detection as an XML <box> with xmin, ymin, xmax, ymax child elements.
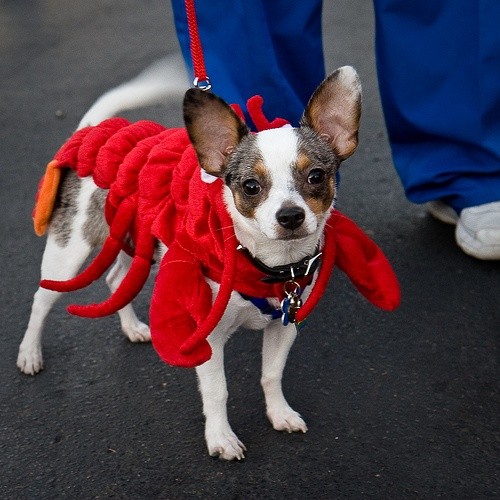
<box><xmin>423</xmin><ymin>195</ymin><xmax>499</xmax><ymax>260</ymax></box>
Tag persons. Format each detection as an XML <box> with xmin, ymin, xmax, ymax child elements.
<box><xmin>170</xmin><ymin>1</ymin><xmax>500</xmax><ymax>261</ymax></box>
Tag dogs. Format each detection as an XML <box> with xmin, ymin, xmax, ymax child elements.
<box><xmin>16</xmin><ymin>53</ymin><xmax>364</xmax><ymax>462</ymax></box>
